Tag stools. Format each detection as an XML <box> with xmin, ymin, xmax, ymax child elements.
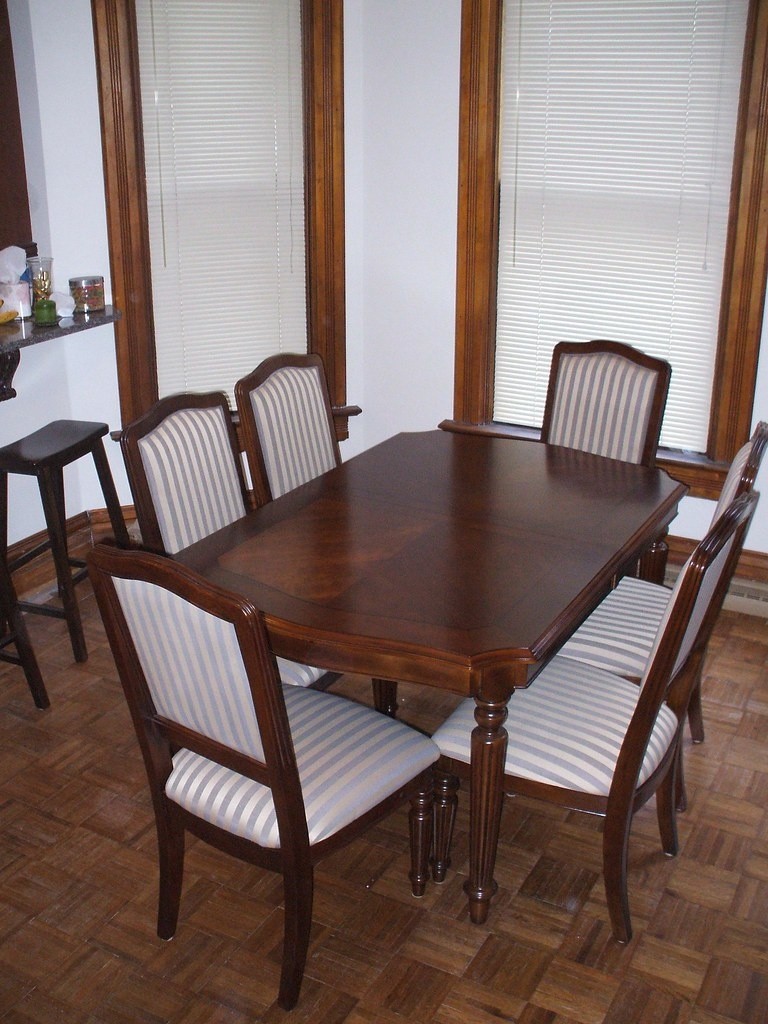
<box><xmin>0</xmin><ymin>420</ymin><xmax>133</xmax><ymax>663</ymax></box>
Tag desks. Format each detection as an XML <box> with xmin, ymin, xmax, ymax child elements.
<box><xmin>170</xmin><ymin>429</ymin><xmax>690</xmax><ymax>924</ymax></box>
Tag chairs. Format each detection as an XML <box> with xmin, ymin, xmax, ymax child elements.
<box><xmin>86</xmin><ymin>543</ymin><xmax>441</xmax><ymax>1012</ymax></box>
<box><xmin>235</xmin><ymin>353</ymin><xmax>342</xmax><ymax>508</ymax></box>
<box><xmin>430</xmin><ymin>490</ymin><xmax>760</xmax><ymax>946</ymax></box>
<box><xmin>556</xmin><ymin>421</ymin><xmax>768</xmax><ymax>812</ymax></box>
<box><xmin>540</xmin><ymin>340</ymin><xmax>672</xmax><ymax>589</ymax></box>
<box><xmin>119</xmin><ymin>391</ymin><xmax>343</xmax><ymax>691</ymax></box>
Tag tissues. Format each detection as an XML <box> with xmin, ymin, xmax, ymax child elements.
<box><xmin>0</xmin><ymin>247</ymin><xmax>32</xmax><ymax>317</ymax></box>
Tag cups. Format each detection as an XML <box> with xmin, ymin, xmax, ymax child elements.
<box><xmin>33</xmin><ymin>300</ymin><xmax>57</xmax><ymax>324</ymax></box>
<box><xmin>25</xmin><ymin>256</ymin><xmax>55</xmax><ymax>300</ymax></box>
<box><xmin>69</xmin><ymin>276</ymin><xmax>105</xmax><ymax>312</ymax></box>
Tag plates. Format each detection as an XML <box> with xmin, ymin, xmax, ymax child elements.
<box><xmin>30</xmin><ymin>316</ymin><xmax>63</xmax><ymax>327</ymax></box>
